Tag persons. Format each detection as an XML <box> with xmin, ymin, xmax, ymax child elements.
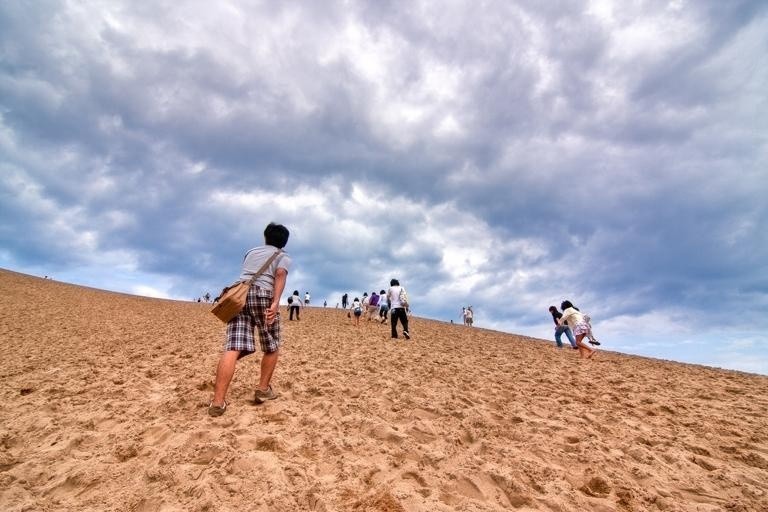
<box><xmin>549</xmin><ymin>306</ymin><xmax>580</xmax><ymax>349</ymax></box>
<box><xmin>208</xmin><ymin>223</ymin><xmax>291</xmax><ymax>416</ymax></box>
<box><xmin>558</xmin><ymin>300</ymin><xmax>596</xmax><ymax>361</ymax></box>
<box><xmin>461</xmin><ymin>305</ymin><xmax>475</xmax><ymax>327</ymax></box>
<box><xmin>197</xmin><ymin>278</ymin><xmax>411</xmax><ymax>338</ymax></box>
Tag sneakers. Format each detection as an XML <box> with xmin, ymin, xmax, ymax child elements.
<box><xmin>381</xmin><ymin>319</ymin><xmax>385</xmax><ymax>324</ymax></box>
<box><xmin>209</xmin><ymin>401</ymin><xmax>226</xmax><ymax>416</ymax></box>
<box><xmin>256</xmin><ymin>386</ymin><xmax>277</xmax><ymax>402</ymax></box>
<box><xmin>403</xmin><ymin>331</ymin><xmax>410</xmax><ymax>339</ymax></box>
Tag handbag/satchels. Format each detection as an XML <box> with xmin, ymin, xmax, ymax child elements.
<box><xmin>288</xmin><ymin>296</ymin><xmax>293</xmax><ymax>303</ymax></box>
<box><xmin>355</xmin><ymin>307</ymin><xmax>360</xmax><ymax>313</ymax></box>
<box><xmin>400</xmin><ymin>291</ymin><xmax>409</xmax><ymax>307</ymax></box>
<box><xmin>211</xmin><ymin>281</ymin><xmax>249</xmax><ymax>322</ymax></box>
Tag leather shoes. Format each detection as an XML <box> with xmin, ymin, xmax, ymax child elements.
<box><xmin>589</xmin><ymin>341</ymin><xmax>600</xmax><ymax>346</ymax></box>
